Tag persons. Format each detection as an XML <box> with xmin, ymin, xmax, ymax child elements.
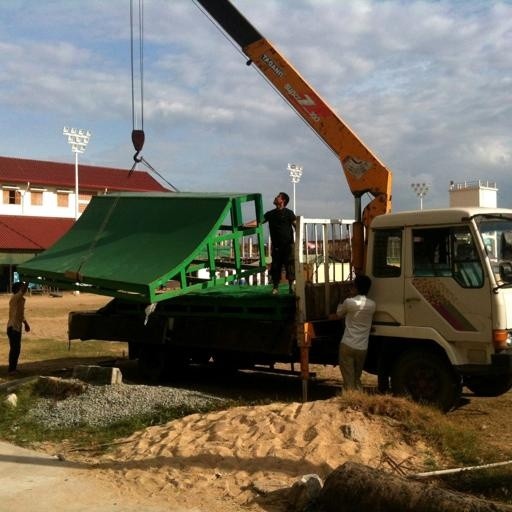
<box><xmin>336</xmin><ymin>276</ymin><xmax>376</xmax><ymax>392</ymax></box>
<box><xmin>244</xmin><ymin>193</ymin><xmax>297</xmax><ymax>295</ymax></box>
<box><xmin>7</xmin><ymin>278</ymin><xmax>30</xmax><ymax>375</ymax></box>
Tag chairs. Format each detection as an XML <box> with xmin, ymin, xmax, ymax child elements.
<box><xmin>412</xmin><ymin>243</ymin><xmax>452</xmax><ymax>276</ymax></box>
<box><xmin>454</xmin><ymin>242</ymin><xmax>483</xmax><ymax>288</ymax></box>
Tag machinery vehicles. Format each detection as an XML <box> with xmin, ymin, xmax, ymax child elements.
<box><xmin>68</xmin><ymin>0</ymin><xmax>512</xmax><ymax>413</ymax></box>
